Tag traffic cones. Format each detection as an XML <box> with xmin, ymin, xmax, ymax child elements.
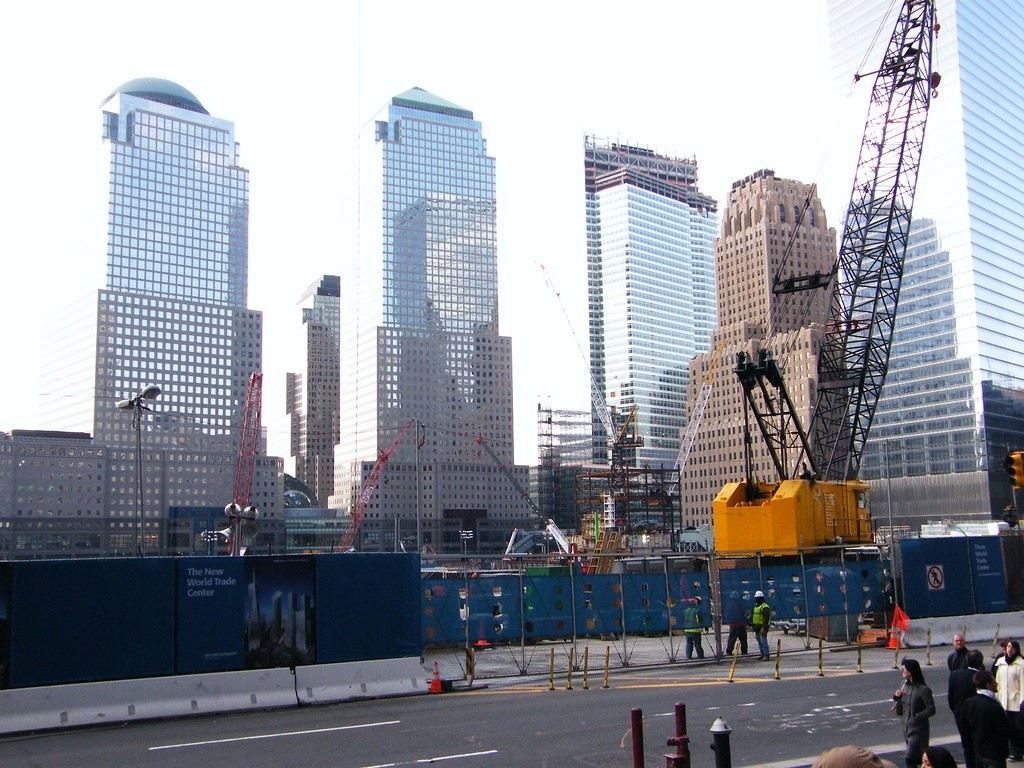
<box><xmin>429</xmin><ymin>661</ymin><xmax>446</xmax><ymax>694</ymax></box>
<box><xmin>888</xmin><ymin>630</ymin><xmax>902</xmax><ymax>650</ymax></box>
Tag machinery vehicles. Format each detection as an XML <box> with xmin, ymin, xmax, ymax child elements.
<box><xmin>229</xmin><ymin>371</ymin><xmax>671</xmax><ymax>559</ymax></box>
<box><xmin>708</xmin><ymin>0</ymin><xmax>941</xmax><ymax>566</ymax></box>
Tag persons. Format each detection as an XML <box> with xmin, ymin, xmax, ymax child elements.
<box><xmin>753</xmin><ymin>591</ymin><xmax>770</xmax><ymax>661</ymax></box>
<box><xmin>725</xmin><ymin>591</ymin><xmax>748</xmax><ymax>654</ymax></box>
<box><xmin>811</xmin><ymin>633</ymin><xmax>1024</xmax><ymax>768</ymax></box>
<box><xmin>684</xmin><ymin>596</ymin><xmax>709</xmax><ymax>659</ymax></box>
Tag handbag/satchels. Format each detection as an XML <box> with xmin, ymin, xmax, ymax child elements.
<box><xmin>890</xmin><ymin>680</ymin><xmax>908</xmax><ymax>716</ymax></box>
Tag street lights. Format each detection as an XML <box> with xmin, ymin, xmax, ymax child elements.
<box><xmin>115</xmin><ymin>385</ymin><xmax>164</xmax><ymax>555</ymax></box>
<box><xmin>458</xmin><ymin>529</ymin><xmax>475</xmax><ymax>685</ymax></box>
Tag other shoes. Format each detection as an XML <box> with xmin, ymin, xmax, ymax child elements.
<box><xmin>757</xmin><ymin>656</ymin><xmax>762</xmax><ymax>660</ymax></box>
<box><xmin>761</xmin><ymin>656</ymin><xmax>769</xmax><ymax>661</ymax></box>
<box><xmin>1008</xmin><ymin>756</ymin><xmax>1023</xmax><ymax>762</ymax></box>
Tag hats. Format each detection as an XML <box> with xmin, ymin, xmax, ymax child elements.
<box><xmin>811</xmin><ymin>746</ymin><xmax>898</xmax><ymax>768</ymax></box>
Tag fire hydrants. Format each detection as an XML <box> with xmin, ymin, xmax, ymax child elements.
<box><xmin>709</xmin><ymin>715</ymin><xmax>733</xmax><ymax>768</ymax></box>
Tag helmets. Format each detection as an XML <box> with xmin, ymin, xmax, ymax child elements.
<box><xmin>754</xmin><ymin>591</ymin><xmax>765</xmax><ymax>597</ymax></box>
<box><xmin>730</xmin><ymin>591</ymin><xmax>741</xmax><ymax>599</ymax></box>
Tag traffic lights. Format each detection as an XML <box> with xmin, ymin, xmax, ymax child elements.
<box><xmin>1005</xmin><ymin>452</ymin><xmax>1024</xmax><ymax>488</ymax></box>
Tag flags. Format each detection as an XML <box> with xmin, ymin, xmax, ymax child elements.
<box><xmin>893</xmin><ymin>604</ymin><xmax>910</xmax><ymax>631</ymax></box>
